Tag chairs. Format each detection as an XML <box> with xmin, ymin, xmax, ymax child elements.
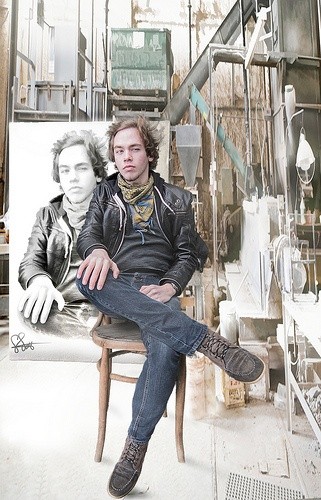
<box><xmin>89</xmin><ymin>286</ymin><xmax>194</xmax><ymax>464</ymax></box>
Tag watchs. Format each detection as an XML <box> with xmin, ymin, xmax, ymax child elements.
<box><xmin>161</xmin><ymin>279</ymin><xmax>180</xmax><ymax>294</ymax></box>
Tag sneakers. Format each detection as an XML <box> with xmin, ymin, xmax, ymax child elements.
<box><xmin>108</xmin><ymin>434</ymin><xmax>148</xmax><ymax>499</ymax></box>
<box><xmin>196</xmin><ymin>328</ymin><xmax>266</xmax><ymax>384</ymax></box>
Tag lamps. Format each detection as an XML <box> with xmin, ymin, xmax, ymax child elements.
<box><xmin>295</xmin><ymin>113</ymin><xmax>315</xmax><ymax>184</ymax></box>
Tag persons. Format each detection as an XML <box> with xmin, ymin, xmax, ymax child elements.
<box><xmin>75</xmin><ymin>113</ymin><xmax>266</xmax><ymax>499</ymax></box>
